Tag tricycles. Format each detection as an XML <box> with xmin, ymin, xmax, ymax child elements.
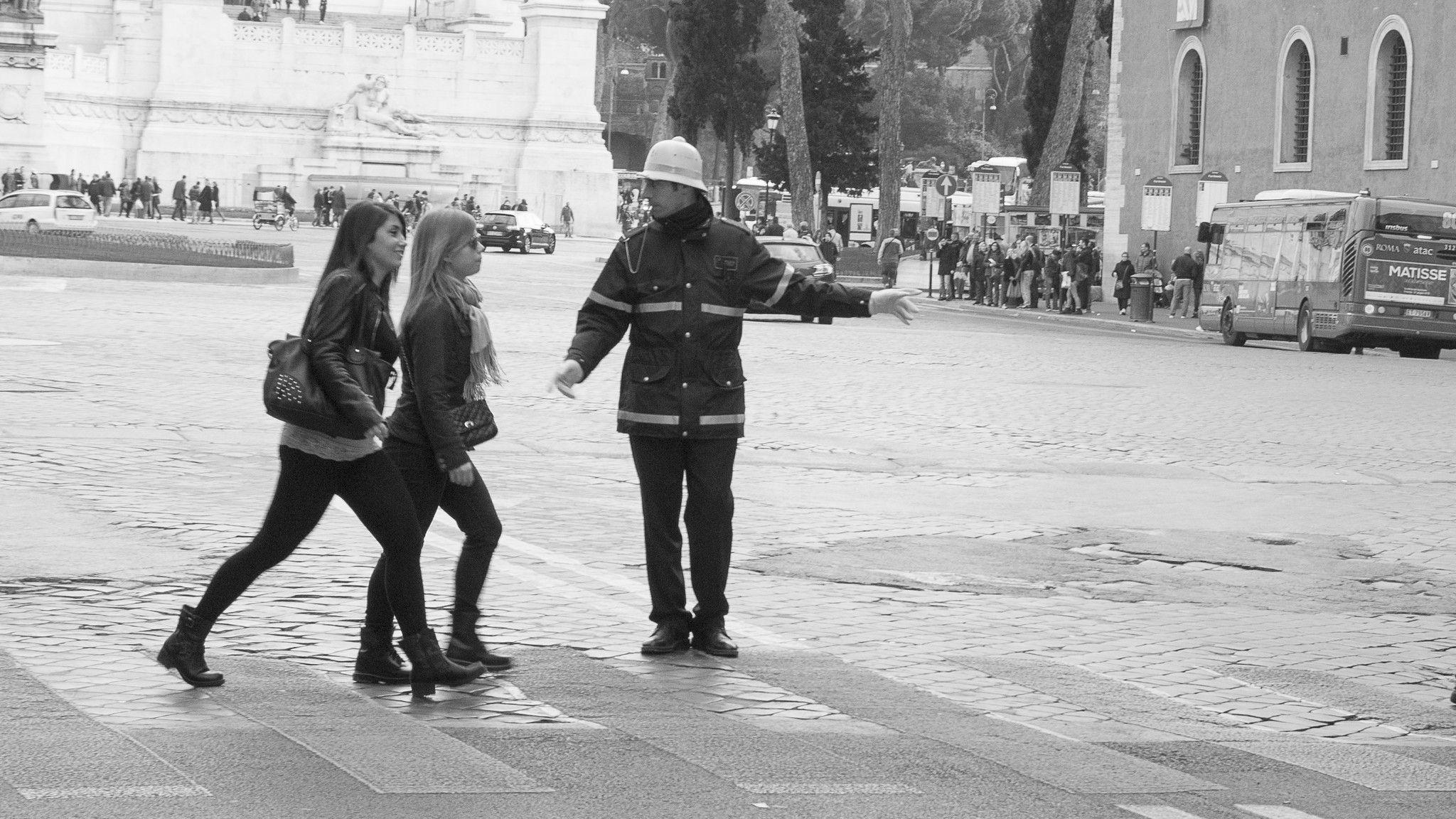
<box><xmin>251</xmin><ymin>186</ymin><xmax>300</xmax><ymax>231</ymax></box>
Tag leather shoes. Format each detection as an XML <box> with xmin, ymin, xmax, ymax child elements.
<box><xmin>692</xmin><ymin>622</ymin><xmax>740</xmax><ymax>657</ymax></box>
<box><xmin>640</xmin><ymin>615</ymin><xmax>690</xmax><ymax>653</ymax></box>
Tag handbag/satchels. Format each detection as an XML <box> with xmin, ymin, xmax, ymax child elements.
<box><xmin>1007</xmin><ymin>278</ymin><xmax>1022</xmax><ymax>298</ymax></box>
<box><xmin>262</xmin><ymin>270</ymin><xmax>398</xmax><ymax>442</ymax></box>
<box><xmin>1115</xmin><ymin>279</ymin><xmax>1123</xmax><ymax>291</ymax></box>
<box><xmin>1060</xmin><ymin>271</ymin><xmax>1072</xmax><ymax>289</ymax></box>
<box><xmin>451</xmin><ymin>399</ymin><xmax>500</xmax><ymax>449</ymax></box>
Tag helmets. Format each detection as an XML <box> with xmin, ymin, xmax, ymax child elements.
<box><xmin>633</xmin><ymin>136</ymin><xmax>708</xmax><ymax>193</ymax></box>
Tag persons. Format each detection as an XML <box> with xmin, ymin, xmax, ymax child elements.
<box><xmin>877</xmin><ymin>229</ymin><xmax>904</xmax><ymax>290</ymax></box>
<box><xmin>353</xmin><ymin>203</ymin><xmax>514</xmax><ymax>688</ymax></box>
<box><xmin>714</xmin><ymin>210</ymin><xmax>843</xmax><ymax>284</ymax></box>
<box><xmin>445</xmin><ymin>194</ymin><xmax>482</xmax><ymax>222</ymax></box>
<box><xmin>556</xmin><ymin>133</ymin><xmax>922</xmax><ymax>653</ymax></box>
<box><xmin>559</xmin><ymin>202</ymin><xmax>574</xmax><ymax>238</ymax></box>
<box><xmin>614</xmin><ymin>184</ymin><xmax>656</xmax><ymax>238</ymax></box>
<box><xmin>501</xmin><ymin>198</ymin><xmax>528</xmax><ymax>212</ymax></box>
<box><xmin>0</xmin><ymin>163</ymin><xmax>226</xmax><ymax>225</ymax></box>
<box><xmin>280</xmin><ymin>187</ymin><xmax>297</xmax><ymax>217</ymax></box>
<box><xmin>338</xmin><ymin>75</ymin><xmax>434</xmax><ymax>139</ymax></box>
<box><xmin>154</xmin><ymin>196</ymin><xmax>487</xmax><ymax>701</ymax></box>
<box><xmin>272</xmin><ymin>185</ymin><xmax>284</xmax><ymax>203</ymax></box>
<box><xmin>311</xmin><ymin>183</ymin><xmax>429</xmax><ymax>232</ymax></box>
<box><xmin>237</xmin><ymin>0</ymin><xmax>328</xmax><ymax>24</ymax></box>
<box><xmin>887</xmin><ymin>228</ymin><xmax>904</xmax><ymax>285</ymax></box>
<box><xmin>931</xmin><ymin>228</ymin><xmax>1204</xmax><ymax>323</ymax></box>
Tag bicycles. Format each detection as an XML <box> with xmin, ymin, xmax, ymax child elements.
<box><xmin>401</xmin><ymin>213</ymin><xmax>419</xmax><ymax>236</ymax></box>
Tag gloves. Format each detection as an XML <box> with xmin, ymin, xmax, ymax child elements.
<box><xmin>868</xmin><ymin>285</ymin><xmax>921</xmax><ymax>327</ymax></box>
<box><xmin>546</xmin><ymin>359</ymin><xmax>584</xmax><ymax>399</ymax></box>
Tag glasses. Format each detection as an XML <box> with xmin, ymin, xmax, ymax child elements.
<box><xmin>455</xmin><ymin>234</ymin><xmax>482</xmax><ymax>253</ymax></box>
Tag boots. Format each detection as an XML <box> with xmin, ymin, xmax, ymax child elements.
<box><xmin>446</xmin><ymin>608</ymin><xmax>512</xmax><ymax>671</ymax></box>
<box><xmin>351</xmin><ymin>621</ymin><xmax>412</xmax><ymax>685</ymax></box>
<box><xmin>397</xmin><ymin>627</ymin><xmax>487</xmax><ymax>696</ymax></box>
<box><xmin>157</xmin><ymin>603</ymin><xmax>225</xmax><ymax>687</ymax></box>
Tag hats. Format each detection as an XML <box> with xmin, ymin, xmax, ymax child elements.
<box><xmin>994</xmin><ymin>235</ymin><xmax>1002</xmax><ymax>240</ymax></box>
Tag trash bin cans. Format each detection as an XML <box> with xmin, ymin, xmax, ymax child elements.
<box><xmin>1129</xmin><ymin>273</ymin><xmax>1155</xmax><ymax>322</ymax></box>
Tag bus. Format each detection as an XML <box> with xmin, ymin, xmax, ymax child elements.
<box><xmin>1198</xmin><ymin>188</ymin><xmax>1456</xmax><ymax>360</ymax></box>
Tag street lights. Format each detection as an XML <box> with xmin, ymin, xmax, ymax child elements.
<box><xmin>981</xmin><ymin>87</ymin><xmax>998</xmax><ymax>161</ymax></box>
<box><xmin>763</xmin><ymin>108</ymin><xmax>780</xmax><ymax>225</ymax></box>
<box><xmin>607</xmin><ymin>49</ymin><xmax>631</xmax><ymax>151</ymax></box>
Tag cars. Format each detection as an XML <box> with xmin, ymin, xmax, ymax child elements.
<box><xmin>476</xmin><ymin>210</ymin><xmax>556</xmax><ymax>254</ymax></box>
<box><xmin>753</xmin><ymin>235</ymin><xmax>837</xmax><ymax>284</ymax></box>
<box><xmin>0</xmin><ymin>189</ymin><xmax>98</xmax><ymax>236</ymax></box>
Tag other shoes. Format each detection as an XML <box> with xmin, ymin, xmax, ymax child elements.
<box><xmin>1192</xmin><ymin>312</ymin><xmax>1198</xmax><ymax>318</ymax></box>
<box><xmin>1169</xmin><ymin>316</ymin><xmax>1174</xmax><ymax>318</ymax></box>
<box><xmin>1181</xmin><ymin>316</ymin><xmax>1185</xmax><ymax>319</ymax></box>
<box><xmin>1119</xmin><ymin>308</ymin><xmax>1126</xmax><ymax>315</ymax></box>
<box><xmin>938</xmin><ymin>295</ymin><xmax>1087</xmax><ymax>314</ymax></box>
